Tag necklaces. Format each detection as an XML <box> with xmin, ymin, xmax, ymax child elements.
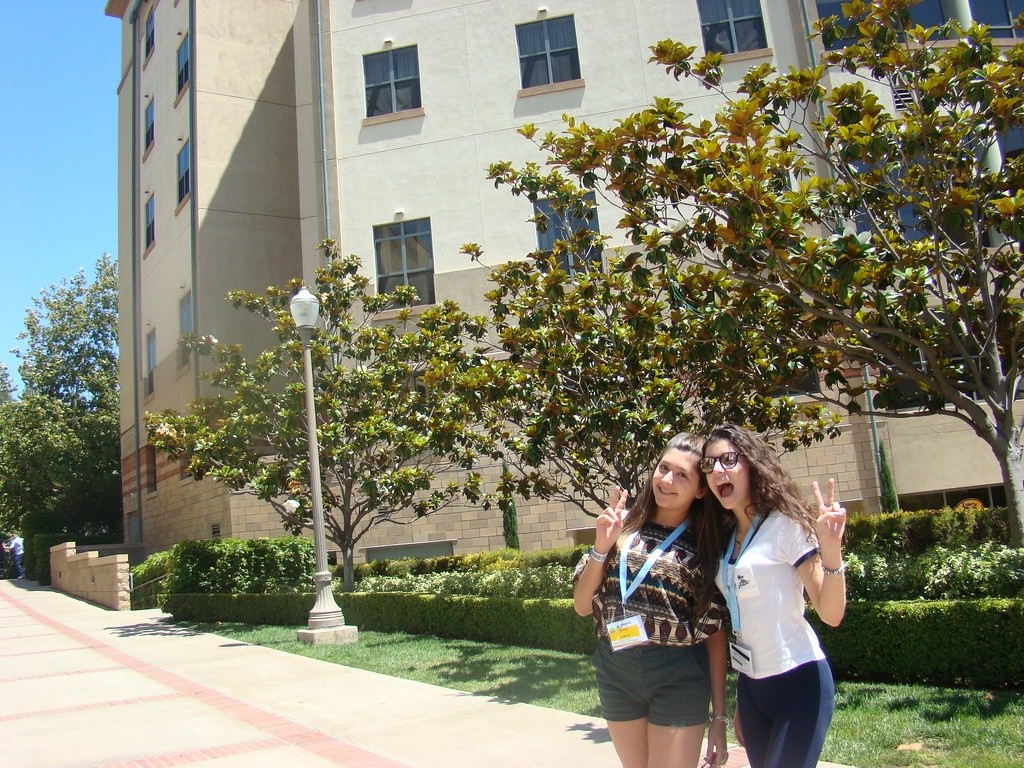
<box><xmin>734</xmin><ymin>541</ymin><xmax>741</xmax><ymax>548</ymax></box>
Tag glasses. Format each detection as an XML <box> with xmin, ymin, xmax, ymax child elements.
<box><xmin>696</xmin><ymin>451</ymin><xmax>744</xmax><ymax>473</ymax></box>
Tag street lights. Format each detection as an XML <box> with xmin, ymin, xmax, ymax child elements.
<box><xmin>288</xmin><ymin>286</ymin><xmax>349</xmax><ymax>629</ymax></box>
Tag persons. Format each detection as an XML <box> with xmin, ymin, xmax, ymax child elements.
<box><xmin>701</xmin><ymin>425</ymin><xmax>848</xmax><ymax>768</ymax></box>
<box><xmin>572</xmin><ymin>432</ymin><xmax>730</xmax><ymax>768</ymax></box>
<box><xmin>3</xmin><ymin>533</ymin><xmax>25</xmax><ymax>578</ymax></box>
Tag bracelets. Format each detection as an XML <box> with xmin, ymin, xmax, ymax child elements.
<box><xmin>713</xmin><ymin>715</ymin><xmax>729</xmax><ymax>724</ymax></box>
<box><xmin>590</xmin><ymin>546</ymin><xmax>608</xmax><ymax>562</ymax></box>
<box><xmin>823</xmin><ymin>562</ymin><xmax>845</xmax><ymax>575</ymax></box>
